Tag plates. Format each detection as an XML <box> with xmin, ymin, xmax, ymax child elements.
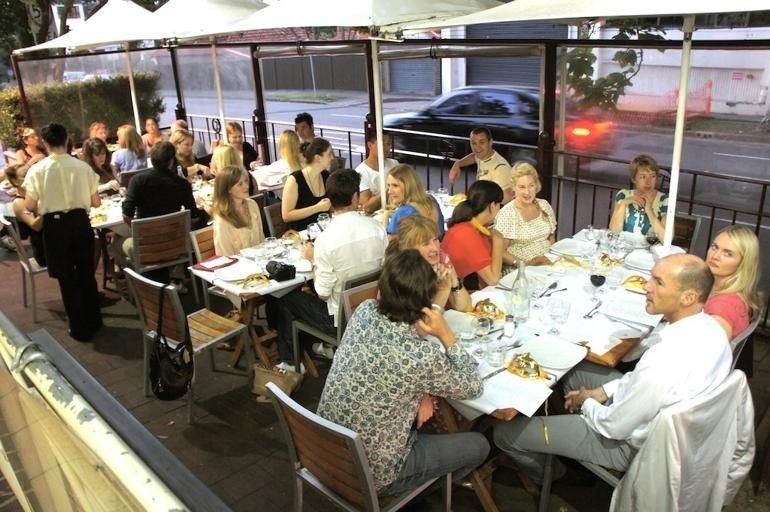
<box><xmin>437</xmin><ymin>225</ymin><xmax>688</xmax><ymax>369</ymax></box>
<box><xmin>438</xmin><ymin>194</ymin><xmax>468</xmax><ymax>209</ymax></box>
<box><xmin>217</xmin><ymin>213</ymin><xmax>331</xmax><ymax>282</ymax></box>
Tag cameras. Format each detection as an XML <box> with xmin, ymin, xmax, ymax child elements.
<box><xmin>266</xmin><ymin>261</ymin><xmax>296</xmax><ymax>282</ymax></box>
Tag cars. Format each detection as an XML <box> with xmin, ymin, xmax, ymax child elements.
<box><xmin>380</xmin><ymin>85</ymin><xmax>619</xmax><ymax>175</ymax></box>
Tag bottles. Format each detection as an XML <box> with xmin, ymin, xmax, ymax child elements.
<box><xmin>176</xmin><ymin>165</ymin><xmax>188</xmax><ymax>179</ymax></box>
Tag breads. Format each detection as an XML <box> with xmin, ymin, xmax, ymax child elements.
<box><xmin>552</xmin><ymin>254</ymin><xmax>582</xmax><ymax>267</ymax></box>
<box><xmin>620</xmin><ymin>275</ymin><xmax>648</xmax><ymax>290</ymax></box>
<box><xmin>470</xmin><ymin>297</ymin><xmax>506</xmax><ymax>320</ymax></box>
<box><xmin>241</xmin><ymin>271</ymin><xmax>269</xmax><ymax>288</ymax></box>
<box><xmin>449</xmin><ymin>193</ymin><xmax>467</xmax><ymax>204</ymax></box>
<box><xmin>282</xmin><ymin>229</ymin><xmax>299</xmax><ymax>242</ymax></box>
<box><xmin>506</xmin><ymin>352</ymin><xmax>547</xmax><ymax>380</ymax></box>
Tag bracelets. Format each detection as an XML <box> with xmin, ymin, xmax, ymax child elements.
<box><xmin>512</xmin><ymin>257</ymin><xmax>528</xmax><ymax>268</ymax></box>
<box><xmin>430</xmin><ymin>303</ymin><xmax>447</xmax><ymax>314</ymax></box>
<box><xmin>450</xmin><ymin>277</ymin><xmax>464</xmax><ymax>292</ymax></box>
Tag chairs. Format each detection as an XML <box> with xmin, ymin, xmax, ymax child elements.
<box><xmin>0</xmin><ymin>153</ymin><xmax>506</xmax><ymax>427</ymax></box>
<box><xmin>263</xmin><ymin>212</ymin><xmax>769</xmax><ymax>511</ymax></box>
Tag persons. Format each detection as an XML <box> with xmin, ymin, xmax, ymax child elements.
<box><xmin>699</xmin><ymin>223</ymin><xmax>760</xmax><ymax>344</ymax></box>
<box><xmin>269</xmin><ymin>165</ymin><xmax>390</xmax><ymax>376</ymax></box>
<box><xmin>280</xmin><ymin>137</ymin><xmax>336</xmax><ymax>232</ymax></box>
<box><xmin>494</xmin><ymin>254</ymin><xmax>733</xmax><ymax>485</ymax></box>
<box><xmin>606</xmin><ymin>151</ymin><xmax>675</xmax><ymax>247</ymax></box>
<box><xmin>384</xmin><ymin>165</ymin><xmax>447</xmax><ymax>246</ymax></box>
<box><xmin>353</xmin><ymin>127</ymin><xmax>401</xmax><ymax>215</ymax></box>
<box><xmin>438</xmin><ymin>179</ymin><xmax>506</xmax><ymax>287</ymax></box>
<box><xmin>0</xmin><ymin>110</ymin><xmax>317</xmax><ymax>345</ymax></box>
<box><xmin>382</xmin><ymin>215</ymin><xmax>473</xmax><ymax>313</ymax></box>
<box><xmin>315</xmin><ymin>248</ymin><xmax>491</xmax><ymax>498</ymax></box>
<box><xmin>449</xmin><ymin>126</ymin><xmax>518</xmax><ymax>210</ymax></box>
<box><xmin>494</xmin><ymin>162</ymin><xmax>559</xmax><ymax>270</ymax></box>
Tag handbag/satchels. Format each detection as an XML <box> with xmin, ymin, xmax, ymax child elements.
<box><xmin>147</xmin><ymin>283</ymin><xmax>196</xmax><ymax>403</ymax></box>
<box><xmin>250</xmin><ymin>364</ymin><xmax>304</xmax><ymax>403</ymax></box>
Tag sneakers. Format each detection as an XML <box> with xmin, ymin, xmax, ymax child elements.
<box><xmin>276</xmin><ymin>362</ymin><xmax>305</xmax><ymax>376</ymax></box>
<box><xmin>312</xmin><ymin>343</ymin><xmax>334</xmax><ymax>360</ymax></box>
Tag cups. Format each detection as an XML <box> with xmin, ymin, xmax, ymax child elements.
<box><xmin>193</xmin><ymin>173</ymin><xmax>204</xmax><ymax>191</ymax></box>
<box><xmin>426</xmin><ymin>189</ymin><xmax>435</xmax><ymax>197</ymax></box>
<box><xmin>357</xmin><ymin>202</ymin><xmax>366</xmax><ymax>216</ymax></box>
<box><xmin>96</xmin><ymin>192</ymin><xmax>122</xmax><ymax>211</ymax></box>
<box><xmin>439</xmin><ymin>187</ymin><xmax>449</xmax><ymax>206</ymax></box>
